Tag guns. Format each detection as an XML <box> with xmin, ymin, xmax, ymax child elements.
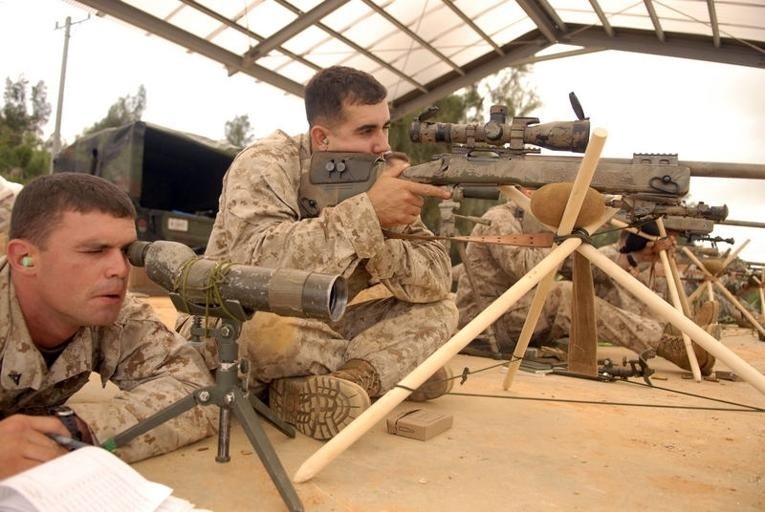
<box><xmin>298</xmin><ymin>92</ymin><xmax>764</xmax><ymax>217</ymax></box>
<box><xmin>682</xmin><ymin>236</ymin><xmax>734</xmax><ymax>249</ymax></box>
<box><xmin>744</xmin><ymin>262</ymin><xmax>765</xmax><ymax>272</ymax></box>
<box><xmin>604</xmin><ymin>199</ymin><xmax>765</xmax><ymax>244</ymax></box>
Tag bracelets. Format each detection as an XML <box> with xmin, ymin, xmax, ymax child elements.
<box><xmin>627</xmin><ymin>254</ymin><xmax>638</xmax><ymax>269</ymax></box>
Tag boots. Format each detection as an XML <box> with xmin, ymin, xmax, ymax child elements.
<box><xmin>695</xmin><ymin>298</ymin><xmax>719</xmax><ymax>327</ymax></box>
<box><xmin>404</xmin><ymin>361</ymin><xmax>455</xmax><ymax>401</ymax></box>
<box><xmin>270</xmin><ymin>359</ymin><xmax>381</xmax><ymax>443</ymax></box>
<box><xmin>657</xmin><ymin>325</ymin><xmax>719</xmax><ymax>376</ymax></box>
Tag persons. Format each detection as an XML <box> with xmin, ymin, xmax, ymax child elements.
<box><xmin>685</xmin><ymin>259</ymin><xmax>765</xmax><ymax>327</ymax></box>
<box><xmin>455</xmin><ymin>184</ymin><xmax>720</xmax><ymax>378</ymax></box>
<box><xmin>0</xmin><ymin>172</ymin><xmax>221</xmax><ymax>480</ymax></box>
<box><xmin>173</xmin><ymin>66</ymin><xmax>457</xmax><ymax>441</ymax></box>
<box><xmin>597</xmin><ymin>220</ymin><xmax>663</xmax><ymax>290</ymax></box>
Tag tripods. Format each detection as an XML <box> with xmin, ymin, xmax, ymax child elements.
<box><xmin>90</xmin><ymin>295</ymin><xmax>307</xmax><ymax>512</ymax></box>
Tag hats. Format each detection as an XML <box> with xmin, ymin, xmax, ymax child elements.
<box><xmin>530</xmin><ymin>182</ymin><xmax>605</xmax><ymax>229</ymax></box>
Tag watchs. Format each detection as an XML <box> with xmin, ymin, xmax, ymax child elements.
<box><xmin>48</xmin><ymin>405</ymin><xmax>83</xmax><ymax>443</ymax></box>
